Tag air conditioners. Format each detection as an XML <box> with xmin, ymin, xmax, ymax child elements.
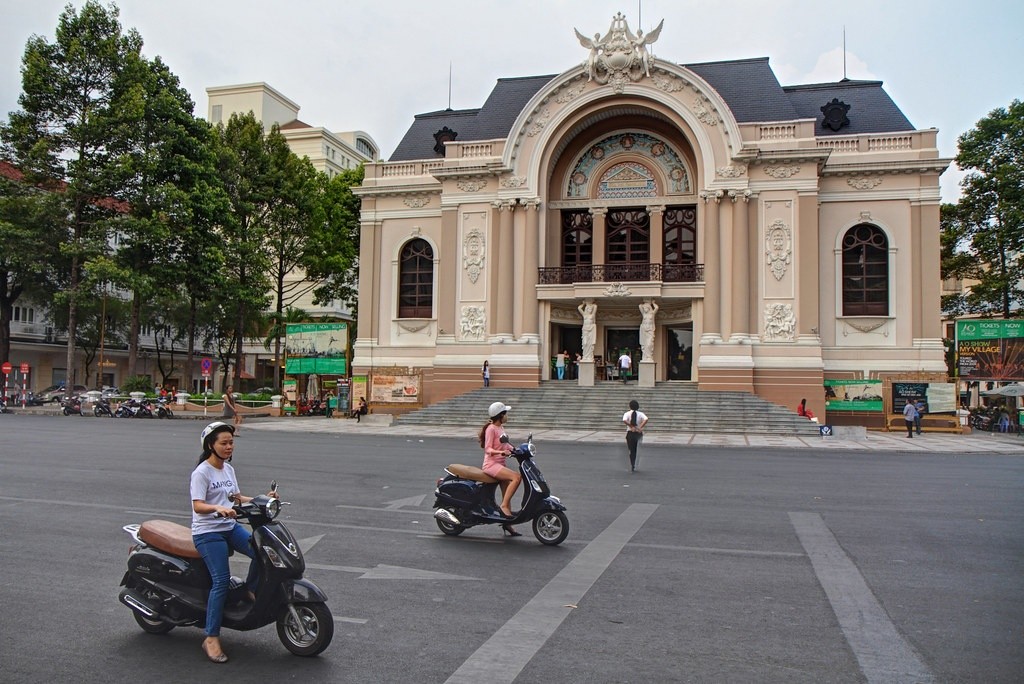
<box><xmin>45</xmin><ymin>326</ymin><xmax>55</xmax><ymax>343</ymax></box>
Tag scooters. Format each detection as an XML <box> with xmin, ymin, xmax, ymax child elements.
<box><xmin>135</xmin><ymin>399</ymin><xmax>154</xmax><ymax>418</ymax></box>
<box><xmin>61</xmin><ymin>393</ymin><xmax>85</xmax><ymax>416</ymax></box>
<box><xmin>94</xmin><ymin>399</ymin><xmax>113</xmax><ymax>417</ymax></box>
<box><xmin>431</xmin><ymin>432</ymin><xmax>570</xmax><ymax>547</ymax></box>
<box><xmin>118</xmin><ymin>478</ymin><xmax>336</xmax><ymax>659</ymax></box>
<box><xmin>115</xmin><ymin>399</ymin><xmax>139</xmax><ymax>419</ymax></box>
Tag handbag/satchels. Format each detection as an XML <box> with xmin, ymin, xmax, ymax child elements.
<box><xmin>920</xmin><ymin>413</ymin><xmax>923</xmax><ymax>418</ymax></box>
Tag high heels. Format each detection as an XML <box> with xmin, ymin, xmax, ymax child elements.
<box><xmin>498</xmin><ymin>507</ymin><xmax>515</xmax><ymax>519</ymax></box>
<box><xmin>502</xmin><ymin>526</ymin><xmax>522</xmax><ymax>536</ymax></box>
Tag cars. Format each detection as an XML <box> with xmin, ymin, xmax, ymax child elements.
<box><xmin>25</xmin><ymin>384</ymin><xmax>89</xmax><ymax>406</ymax></box>
<box><xmin>71</xmin><ymin>389</ymin><xmax>127</xmax><ymax>407</ymax></box>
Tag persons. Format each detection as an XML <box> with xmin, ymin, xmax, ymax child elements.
<box><xmin>903</xmin><ymin>397</ymin><xmax>915</xmax><ymax>437</ymax></box>
<box><xmin>282</xmin><ymin>392</ymin><xmax>288</xmax><ymax>404</ymax></box>
<box><xmin>190</xmin><ymin>422</ymin><xmax>279</xmax><ymax>664</ymax></box>
<box><xmin>617</xmin><ymin>351</ymin><xmax>631</xmax><ymax>384</ymax></box>
<box><xmin>555</xmin><ymin>350</ymin><xmax>581</xmax><ymax>380</ymax></box>
<box><xmin>912</xmin><ymin>398</ymin><xmax>925</xmax><ymax>435</ymax></box>
<box><xmin>482</xmin><ymin>360</ymin><xmax>489</xmax><ymax>388</ymax></box>
<box><xmin>798</xmin><ymin>399</ymin><xmax>811</xmax><ymax>420</ymax></box>
<box><xmin>639</xmin><ymin>299</ymin><xmax>658</xmax><ymax>361</ymax></box>
<box><xmin>326</xmin><ymin>390</ymin><xmax>334</xmax><ymax>417</ymax></box>
<box><xmin>350</xmin><ymin>397</ymin><xmax>367</xmax><ymax>422</ymax></box>
<box><xmin>155</xmin><ymin>382</ymin><xmax>173</xmax><ymax>396</ymax></box>
<box><xmin>460</xmin><ymin>305</ymin><xmax>487</xmax><ymax>339</ymax></box>
<box><xmin>623</xmin><ymin>400</ymin><xmax>648</xmax><ymax>471</ymax></box>
<box><xmin>187</xmin><ymin>384</ymin><xmax>195</xmax><ymax>393</ymax></box>
<box><xmin>764</xmin><ymin>303</ymin><xmax>796</xmax><ymax>336</ymax></box>
<box><xmin>587</xmin><ymin>33</ymin><xmax>607</xmax><ymax>82</ymax></box>
<box><xmin>222</xmin><ymin>385</ymin><xmax>240</xmax><ymax>436</ymax></box>
<box><xmin>477</xmin><ymin>402</ymin><xmax>523</xmax><ymax>536</ymax></box>
<box><xmin>669</xmin><ymin>363</ymin><xmax>678</xmax><ymax>380</ymax></box>
<box><xmin>577</xmin><ymin>300</ymin><xmax>597</xmax><ymax>360</ymax></box>
<box><xmin>962</xmin><ymin>401</ymin><xmax>966</xmax><ymax>410</ymax></box>
<box><xmin>631</xmin><ymin>29</ymin><xmax>650</xmax><ymax>77</ymax></box>
<box><xmin>986</xmin><ymin>408</ymin><xmax>1010</xmax><ymax>433</ymax></box>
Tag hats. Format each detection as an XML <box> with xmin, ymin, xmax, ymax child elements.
<box><xmin>489</xmin><ymin>402</ymin><xmax>511</xmax><ymax>417</ymax></box>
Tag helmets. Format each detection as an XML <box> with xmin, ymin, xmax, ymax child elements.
<box><xmin>201</xmin><ymin>422</ymin><xmax>228</xmax><ymax>450</ymax></box>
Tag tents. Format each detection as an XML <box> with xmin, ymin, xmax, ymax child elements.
<box><xmin>984</xmin><ymin>383</ymin><xmax>1024</xmax><ymax>399</ymax></box>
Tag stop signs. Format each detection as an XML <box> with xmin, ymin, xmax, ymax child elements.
<box><xmin>1</xmin><ymin>361</ymin><xmax>12</xmax><ymax>374</ymax></box>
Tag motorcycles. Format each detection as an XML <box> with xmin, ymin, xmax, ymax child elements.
<box><xmin>156</xmin><ymin>395</ymin><xmax>174</xmax><ymax>420</ymax></box>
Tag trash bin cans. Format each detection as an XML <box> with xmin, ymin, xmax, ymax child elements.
<box><xmin>86</xmin><ymin>391</ymin><xmax>102</xmax><ymax>410</ymax></box>
<box><xmin>1016</xmin><ymin>409</ymin><xmax>1024</xmax><ymax>433</ymax></box>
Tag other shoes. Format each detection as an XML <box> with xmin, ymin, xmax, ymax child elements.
<box><xmin>201</xmin><ymin>641</ymin><xmax>228</xmax><ymax>663</ymax></box>
